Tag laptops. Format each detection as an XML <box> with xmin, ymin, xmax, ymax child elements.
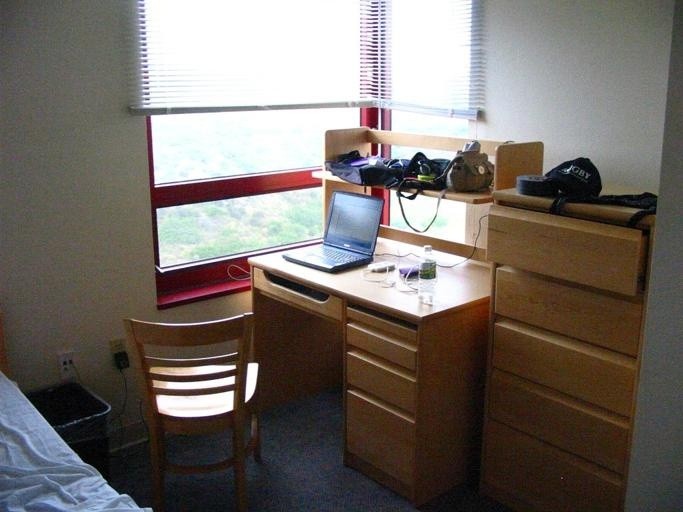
<box><xmin>281</xmin><ymin>190</ymin><xmax>386</xmax><ymax>273</ymax></box>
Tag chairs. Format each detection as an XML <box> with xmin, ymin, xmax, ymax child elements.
<box><xmin>122</xmin><ymin>311</ymin><xmax>260</xmax><ymax>512</ymax></box>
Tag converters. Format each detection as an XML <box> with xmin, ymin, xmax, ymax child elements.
<box><xmin>114</xmin><ymin>351</ymin><xmax>130</xmax><ymax>370</ymax></box>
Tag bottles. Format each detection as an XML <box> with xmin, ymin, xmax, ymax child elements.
<box><xmin>418</xmin><ymin>245</ymin><xmax>437</xmax><ymax>306</ymax></box>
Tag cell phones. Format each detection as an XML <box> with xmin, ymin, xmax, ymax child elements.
<box><xmin>369</xmin><ymin>260</ymin><xmax>395</xmax><ymax>273</ymax></box>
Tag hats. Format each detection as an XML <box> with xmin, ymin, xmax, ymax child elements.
<box><xmin>545</xmin><ymin>157</ymin><xmax>603</xmax><ymax>196</ymax></box>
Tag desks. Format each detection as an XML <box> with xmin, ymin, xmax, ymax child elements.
<box><xmin>247</xmin><ymin>125</ymin><xmax>544</xmax><ymax>508</ymax></box>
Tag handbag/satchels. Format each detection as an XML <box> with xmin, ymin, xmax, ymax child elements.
<box><xmin>443</xmin><ymin>149</ymin><xmax>494</xmax><ymax>194</ymax></box>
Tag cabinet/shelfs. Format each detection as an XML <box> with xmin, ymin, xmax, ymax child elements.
<box><xmin>478</xmin><ymin>183</ymin><xmax>657</xmax><ymax>509</ymax></box>
